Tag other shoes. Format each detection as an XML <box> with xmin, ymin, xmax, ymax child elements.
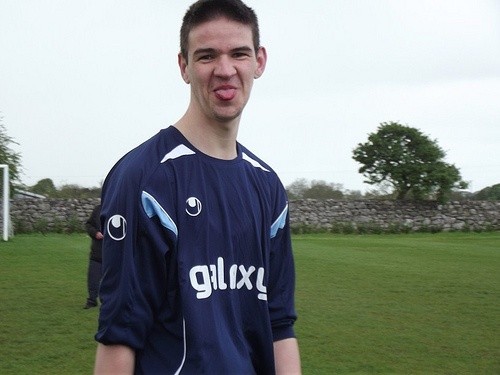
<box><xmin>83</xmin><ymin>298</ymin><xmax>97</xmax><ymax>309</ymax></box>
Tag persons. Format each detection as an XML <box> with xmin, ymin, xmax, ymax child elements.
<box><xmin>83</xmin><ymin>204</ymin><xmax>104</xmax><ymax>310</ymax></box>
<box><xmin>93</xmin><ymin>1</ymin><xmax>302</xmax><ymax>375</ymax></box>
